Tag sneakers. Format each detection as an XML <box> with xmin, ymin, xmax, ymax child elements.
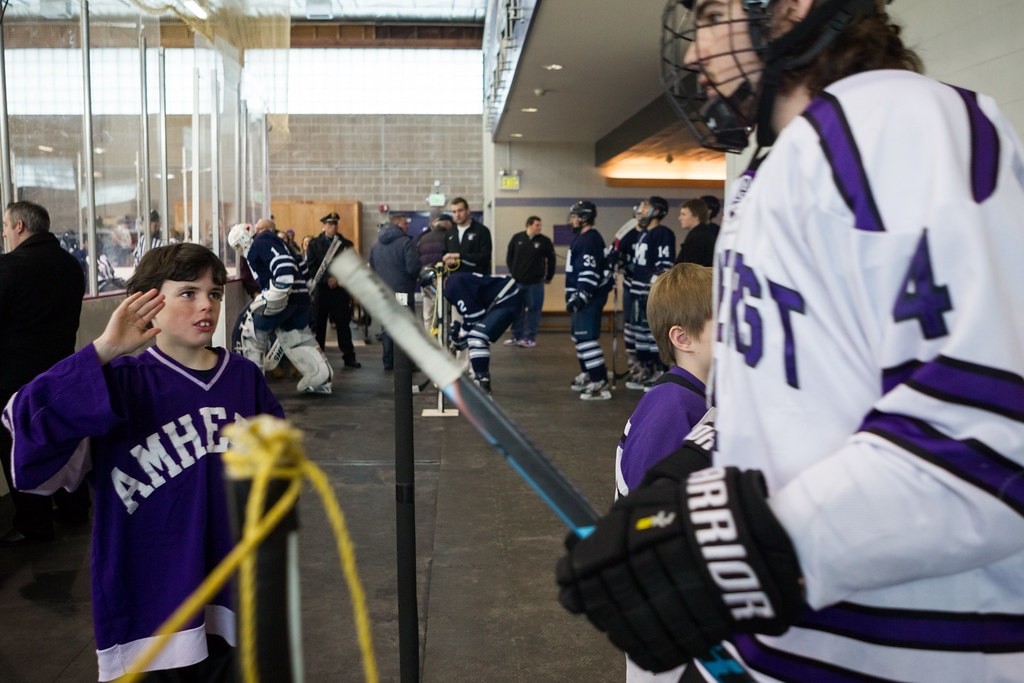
<box><xmin>643</xmin><ymin>372</ymin><xmax>668</xmax><ymax>392</ymax></box>
<box><xmin>503</xmin><ymin>338</ymin><xmax>518</xmax><ymax>347</ymax></box>
<box><xmin>570</xmin><ymin>372</ymin><xmax>592</xmax><ymax>392</ymax></box>
<box><xmin>625</xmin><ymin>365</ymin><xmax>653</xmax><ymax>389</ymax></box>
<box><xmin>518</xmin><ymin>338</ymin><xmax>537</xmax><ymax>347</ymax></box>
<box><xmin>580</xmin><ymin>382</ymin><xmax>612</xmax><ymax>400</ymax></box>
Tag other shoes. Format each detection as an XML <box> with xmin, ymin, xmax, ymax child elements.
<box><xmin>343</xmin><ymin>360</ymin><xmax>362</xmax><ymax>368</ymax></box>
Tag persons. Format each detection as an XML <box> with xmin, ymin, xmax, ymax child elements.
<box><xmin>415</xmin><ymin>214</ymin><xmax>452</xmax><ymax>336</ymax></box>
<box><xmin>369</xmin><ymin>215</ymin><xmax>421</xmax><ymax>372</ymax></box>
<box><xmin>306</xmin><ymin>213</ymin><xmax>361</xmax><ymax>368</ymax></box>
<box><xmin>699</xmin><ymin>195</ymin><xmax>720</xmax><ymax>235</ymax></box>
<box><xmin>563</xmin><ymin>196</ymin><xmax>676</xmax><ymax>400</ymax></box>
<box><xmin>614</xmin><ymin>261</ymin><xmax>713</xmax><ymax>683</ymax></box>
<box><xmin>444</xmin><ymin>197</ymin><xmax>493</xmax><ymax>276</ymax></box>
<box><xmin>132</xmin><ymin>210</ymin><xmax>163</xmax><ymax>268</ymax></box>
<box><xmin>675</xmin><ymin>199</ymin><xmax>718</xmax><ymax>267</ymax></box>
<box><xmin>228</xmin><ymin>218</ymin><xmax>333</xmax><ymax>394</ymax></box>
<box><xmin>0</xmin><ymin>200</ymin><xmax>85</xmax><ymax>603</ymax></box>
<box><xmin>63</xmin><ymin>230</ymin><xmax>115</xmax><ymax>294</ymax></box>
<box><xmin>94</xmin><ymin>213</ymin><xmax>236</xmax><ymax>268</ymax></box>
<box><xmin>417</xmin><ymin>264</ymin><xmax>522</xmax><ymax>402</ymax></box>
<box><xmin>503</xmin><ymin>216</ymin><xmax>556</xmax><ymax>347</ymax></box>
<box><xmin>0</xmin><ymin>242</ymin><xmax>297</xmax><ymax>683</ymax></box>
<box><xmin>554</xmin><ymin>0</ymin><xmax>1024</xmax><ymax>683</ymax></box>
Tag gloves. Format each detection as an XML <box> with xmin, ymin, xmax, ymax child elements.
<box><xmin>556</xmin><ymin>467</ymin><xmax>805</xmax><ymax>675</ymax></box>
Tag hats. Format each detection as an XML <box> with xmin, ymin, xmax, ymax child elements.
<box><xmin>320</xmin><ymin>212</ymin><xmax>340</xmax><ymax>221</ymax></box>
<box><xmin>389</xmin><ymin>210</ymin><xmax>418</xmax><ymax>220</ymax></box>
<box><xmin>433</xmin><ymin>214</ymin><xmax>454</xmax><ymax>222</ymax></box>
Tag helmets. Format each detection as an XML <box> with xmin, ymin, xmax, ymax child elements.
<box><xmin>416</xmin><ymin>262</ymin><xmax>448</xmax><ymax>296</ymax></box>
<box><xmin>569</xmin><ymin>201</ymin><xmax>596</xmax><ymax>232</ymax></box>
<box><xmin>228</xmin><ymin>224</ymin><xmax>253</xmax><ymax>247</ymax></box>
<box><xmin>636</xmin><ymin>196</ymin><xmax>669</xmax><ymax>228</ymax></box>
